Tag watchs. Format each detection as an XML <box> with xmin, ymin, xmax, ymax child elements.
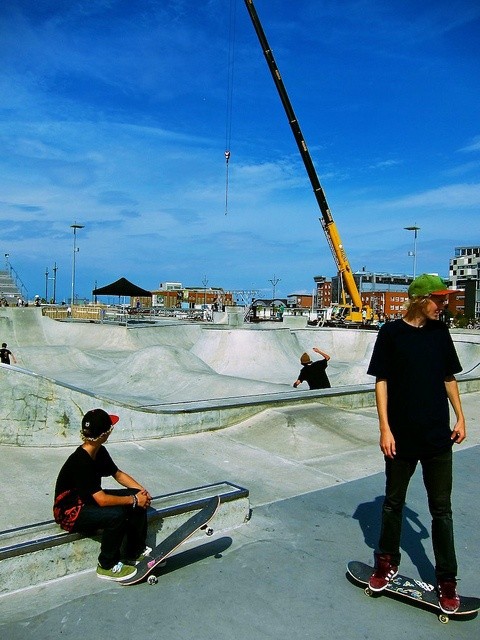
<box><xmin>132</xmin><ymin>495</ymin><xmax>138</xmax><ymax>507</ymax></box>
<box><xmin>53</xmin><ymin>409</ymin><xmax>152</xmax><ymax>582</ymax></box>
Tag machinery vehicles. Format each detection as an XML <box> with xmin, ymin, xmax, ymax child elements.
<box><xmin>224</xmin><ymin>1</ymin><xmax>381</xmax><ymax>329</ymax></box>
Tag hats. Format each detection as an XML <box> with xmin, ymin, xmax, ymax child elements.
<box><xmin>80</xmin><ymin>409</ymin><xmax>118</xmax><ymax>442</ymax></box>
<box><xmin>301</xmin><ymin>353</ymin><xmax>311</xmax><ymax>364</ymax></box>
<box><xmin>406</xmin><ymin>273</ymin><xmax>462</xmax><ymax>298</ymax></box>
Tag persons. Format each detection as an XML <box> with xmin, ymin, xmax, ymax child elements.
<box><xmin>0</xmin><ymin>343</ymin><xmax>17</xmax><ymax>365</ymax></box>
<box><xmin>137</xmin><ymin>299</ymin><xmax>140</xmax><ymax>308</ymax></box>
<box><xmin>331</xmin><ymin>308</ymin><xmax>388</xmax><ymax>325</ymax></box>
<box><xmin>0</xmin><ymin>295</ymin><xmax>71</xmax><ymax>317</ymax></box>
<box><xmin>293</xmin><ymin>347</ymin><xmax>331</xmax><ymax>390</ymax></box>
<box><xmin>367</xmin><ymin>275</ymin><xmax>466</xmax><ymax>613</ymax></box>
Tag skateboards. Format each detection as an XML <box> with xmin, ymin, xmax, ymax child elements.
<box><xmin>346</xmin><ymin>560</ymin><xmax>480</xmax><ymax>624</ymax></box>
<box><xmin>118</xmin><ymin>495</ymin><xmax>220</xmax><ymax>586</ymax></box>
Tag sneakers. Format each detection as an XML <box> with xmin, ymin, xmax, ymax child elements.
<box><xmin>96</xmin><ymin>563</ymin><xmax>138</xmax><ymax>581</ymax></box>
<box><xmin>437</xmin><ymin>578</ymin><xmax>460</xmax><ymax>614</ymax></box>
<box><xmin>124</xmin><ymin>547</ymin><xmax>167</xmax><ymax>568</ymax></box>
<box><xmin>368</xmin><ymin>552</ymin><xmax>400</xmax><ymax>592</ymax></box>
